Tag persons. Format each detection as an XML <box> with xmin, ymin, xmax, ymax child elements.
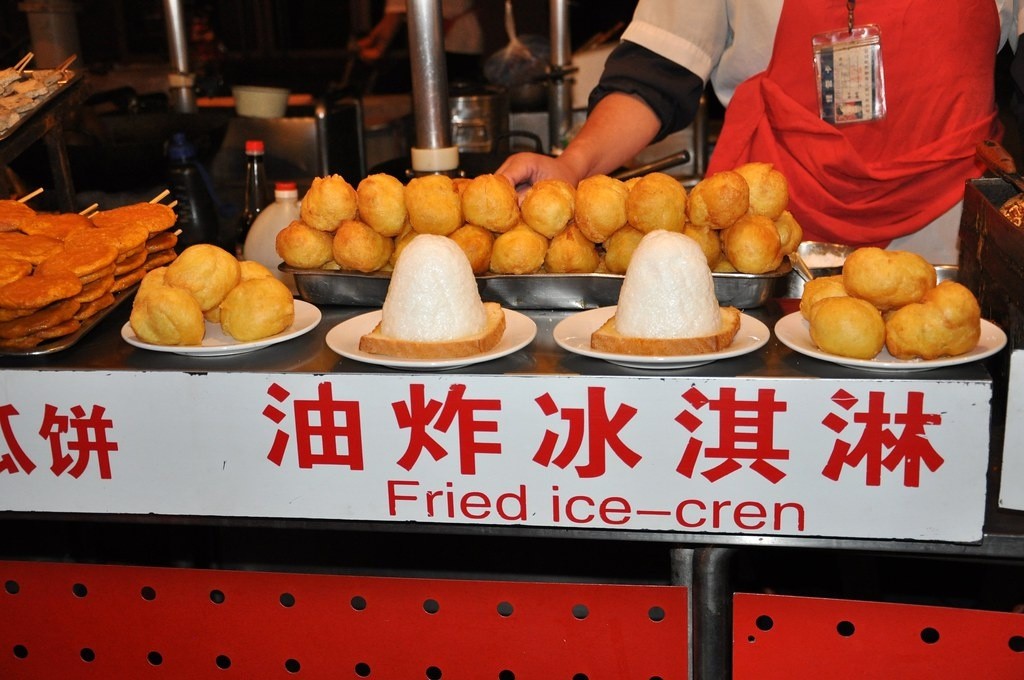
<box><xmin>836</xmin><ymin>101</ymin><xmax>863</xmax><ymax>119</ymax></box>
<box><xmin>356</xmin><ymin>0</ymin><xmax>485</xmax><ymax>80</ymax></box>
<box><xmin>493</xmin><ymin>0</ymin><xmax>1024</xmax><ymax>265</ymax></box>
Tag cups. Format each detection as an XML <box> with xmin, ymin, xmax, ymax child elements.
<box><xmin>449</xmin><ymin>94</ymin><xmax>497</xmax><ymax>153</ymax></box>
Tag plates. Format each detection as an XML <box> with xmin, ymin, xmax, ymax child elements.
<box><xmin>120</xmin><ymin>300</ymin><xmax>321</xmax><ymax>357</ymax></box>
<box><xmin>325</xmin><ymin>311</ymin><xmax>539</xmax><ymax>368</ymax></box>
<box><xmin>554</xmin><ymin>304</ymin><xmax>771</xmax><ymax>369</ymax></box>
<box><xmin>775</xmin><ymin>311</ymin><xmax>1009</xmax><ymax>373</ymax></box>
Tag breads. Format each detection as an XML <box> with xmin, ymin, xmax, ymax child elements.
<box><xmin>130</xmin><ymin>245</ymin><xmax>294</xmax><ymax>342</ymax></box>
<box><xmin>275</xmin><ymin>162</ymin><xmax>982</xmax><ymax>360</ymax></box>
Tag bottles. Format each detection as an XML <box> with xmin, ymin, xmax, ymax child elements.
<box><xmin>152</xmin><ymin>75</ymin><xmax>309</xmax><ymax>295</ymax></box>
<box><xmin>501</xmin><ymin>82</ymin><xmax>555</xmax><ymax>155</ymax></box>
